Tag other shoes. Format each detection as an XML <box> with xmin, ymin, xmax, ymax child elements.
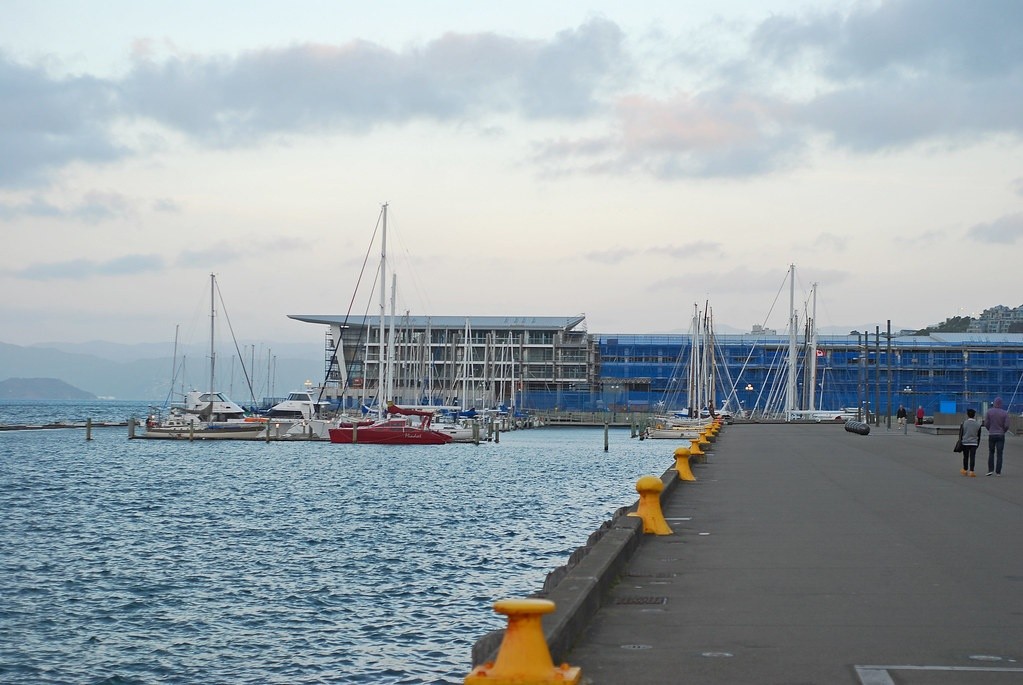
<box><xmin>960</xmin><ymin>469</ymin><xmax>967</xmax><ymax>476</ymax></box>
<box><xmin>970</xmin><ymin>471</ymin><xmax>975</xmax><ymax>477</ymax></box>
<box><xmin>997</xmin><ymin>473</ymin><xmax>1000</xmax><ymax>477</ymax></box>
<box><xmin>985</xmin><ymin>471</ymin><xmax>994</xmax><ymax>475</ymax></box>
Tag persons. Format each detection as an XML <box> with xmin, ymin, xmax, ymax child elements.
<box><xmin>897</xmin><ymin>405</ymin><xmax>906</xmax><ymax>430</ymax></box>
<box><xmin>984</xmin><ymin>397</ymin><xmax>1008</xmax><ymax>476</ymax></box>
<box><xmin>917</xmin><ymin>406</ymin><xmax>924</xmax><ymax>425</ymax></box>
<box><xmin>960</xmin><ymin>408</ymin><xmax>981</xmax><ymax>476</ymax></box>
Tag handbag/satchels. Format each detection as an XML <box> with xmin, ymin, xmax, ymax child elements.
<box><xmin>953</xmin><ymin>439</ymin><xmax>962</xmax><ymax>453</ymax></box>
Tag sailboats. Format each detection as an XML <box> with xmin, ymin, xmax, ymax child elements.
<box><xmin>643</xmin><ymin>262</ymin><xmax>868</xmax><ymax>439</ymax></box>
<box><xmin>146</xmin><ymin>200</ymin><xmax>546</xmax><ymax>446</ymax></box>
